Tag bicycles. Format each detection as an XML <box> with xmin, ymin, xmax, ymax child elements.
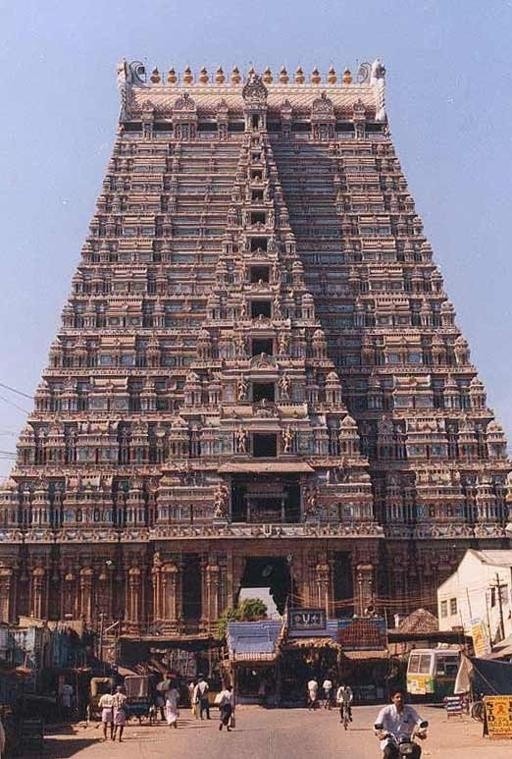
<box><xmin>468</xmin><ymin>691</ymin><xmax>487</xmax><ymax>724</ymax></box>
<box><xmin>336</xmin><ymin>698</ymin><xmax>353</xmax><ymax>729</ymax></box>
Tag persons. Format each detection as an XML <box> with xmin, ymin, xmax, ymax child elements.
<box><xmin>217</xmin><ymin>684</ymin><xmax>235</xmax><ymax>732</ymax></box>
<box><xmin>335</xmin><ymin>681</ymin><xmax>354</xmax><ymax>723</ymax></box>
<box><xmin>97</xmin><ymin>688</ymin><xmax>114</xmax><ymax>741</ymax></box>
<box><xmin>111</xmin><ymin>685</ymin><xmax>127</xmax><ymax>743</ymax></box>
<box><xmin>156</xmin><ymin>673</ymin><xmax>212</xmax><ymax>729</ymax></box>
<box><xmin>322</xmin><ymin>677</ymin><xmax>333</xmax><ymax>711</ymax></box>
<box><xmin>373</xmin><ymin>689</ymin><xmax>428</xmax><ymax>759</ymax></box>
<box><xmin>61</xmin><ymin>682</ymin><xmax>74</xmax><ymax>722</ymax></box>
<box><xmin>307</xmin><ymin>675</ymin><xmax>318</xmax><ymax>712</ymax></box>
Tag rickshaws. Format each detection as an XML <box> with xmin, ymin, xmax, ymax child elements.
<box><xmin>121</xmin><ymin>672</ymin><xmax>158</xmax><ymax>727</ymax></box>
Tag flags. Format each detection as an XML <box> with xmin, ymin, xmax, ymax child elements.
<box><xmin>452</xmin><ymin>653</ymin><xmax>475</xmax><ymax>695</ymax></box>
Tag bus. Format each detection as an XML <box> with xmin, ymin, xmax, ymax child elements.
<box><xmin>407</xmin><ymin>647</ymin><xmax>465</xmax><ymax>704</ymax></box>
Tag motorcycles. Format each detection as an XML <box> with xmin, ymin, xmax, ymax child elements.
<box><xmin>373</xmin><ymin>719</ymin><xmax>430</xmax><ymax>759</ymax></box>
